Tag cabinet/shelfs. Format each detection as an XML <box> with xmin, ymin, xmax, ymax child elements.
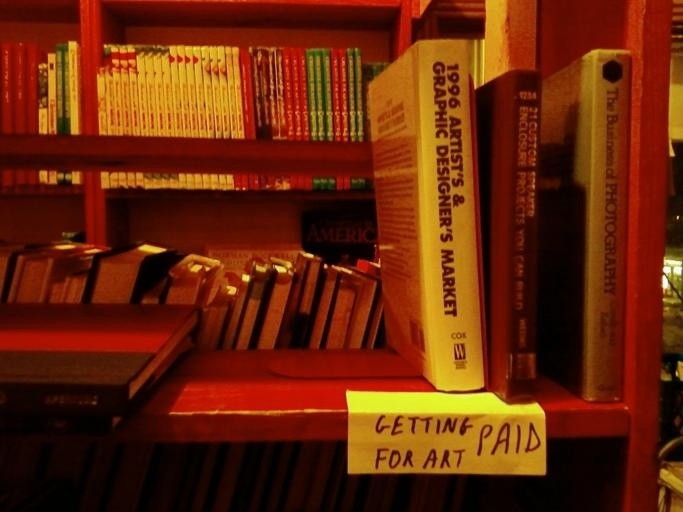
<box><xmin>0</xmin><ymin>0</ymin><xmax>673</xmax><ymax>512</ymax></box>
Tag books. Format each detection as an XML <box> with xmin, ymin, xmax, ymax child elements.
<box><xmin>543</xmin><ymin>48</ymin><xmax>632</xmax><ymax>403</ymax></box>
<box><xmin>1</xmin><ymin>170</ymin><xmax>372</xmax><ymax>191</ymax></box>
<box><xmin>0</xmin><ymin>238</ymin><xmax>475</xmax><ymax>511</ymax></box>
<box><xmin>368</xmin><ymin>41</ymin><xmax>485</xmax><ymax>395</ymax></box>
<box><xmin>1</xmin><ymin>39</ymin><xmax>390</xmax><ymax>141</ymax></box>
<box><xmin>473</xmin><ymin>68</ymin><xmax>542</xmax><ymax>404</ymax></box>
